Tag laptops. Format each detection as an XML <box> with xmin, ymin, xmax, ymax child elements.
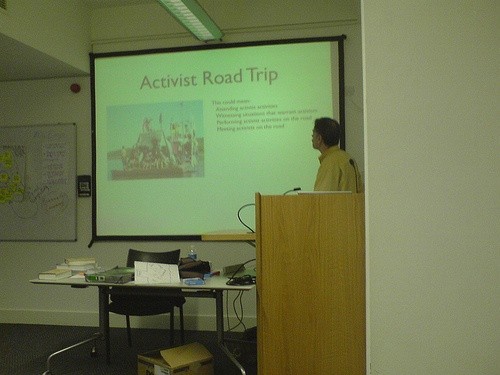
<box><xmin>298</xmin><ymin>191</ymin><xmax>352</xmax><ymax>193</ymax></box>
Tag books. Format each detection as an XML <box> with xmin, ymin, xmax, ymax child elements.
<box><xmin>38</xmin><ymin>256</ymin><xmax>98</xmax><ymax>281</ymax></box>
<box><xmin>183</xmin><ymin>278</ymin><xmax>204</xmax><ymax>285</ymax></box>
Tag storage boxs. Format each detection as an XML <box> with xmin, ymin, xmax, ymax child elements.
<box><xmin>137</xmin><ymin>341</ymin><xmax>215</xmax><ymax>375</ymax></box>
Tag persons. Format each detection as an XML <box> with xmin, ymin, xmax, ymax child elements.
<box><xmin>311</xmin><ymin>117</ymin><xmax>362</xmax><ymax>193</ymax></box>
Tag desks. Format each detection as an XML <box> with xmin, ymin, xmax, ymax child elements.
<box><xmin>27</xmin><ymin>265</ymin><xmax>256</xmax><ymax>375</ymax></box>
<box><xmin>256</xmin><ymin>191</ymin><xmax>365</xmax><ymax>375</ymax></box>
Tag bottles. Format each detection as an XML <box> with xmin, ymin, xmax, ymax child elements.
<box><xmin>188</xmin><ymin>244</ymin><xmax>197</xmax><ymax>260</ymax></box>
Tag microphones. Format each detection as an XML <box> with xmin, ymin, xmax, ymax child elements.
<box><xmin>349</xmin><ymin>159</ymin><xmax>358</xmax><ymax>193</ymax></box>
<box><xmin>283</xmin><ymin>187</ymin><xmax>301</xmax><ymax>194</ymax></box>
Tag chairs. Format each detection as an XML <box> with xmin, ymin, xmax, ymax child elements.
<box><xmin>108</xmin><ymin>248</ymin><xmax>185</xmax><ymax>351</ymax></box>
